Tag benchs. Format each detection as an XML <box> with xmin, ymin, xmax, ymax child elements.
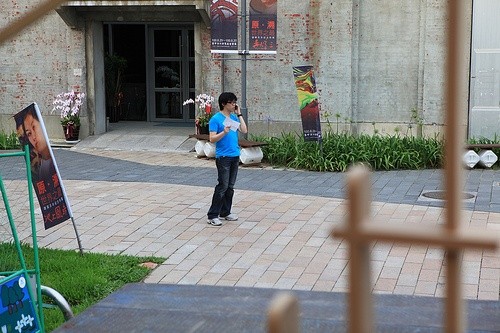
<box><xmin>189</xmin><ymin>134</ymin><xmax>270</xmax><ymax>167</ymax></box>
<box><xmin>464</xmin><ymin>144</ymin><xmax>500</xmax><ymax>168</ymax></box>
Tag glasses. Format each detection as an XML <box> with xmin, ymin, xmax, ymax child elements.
<box><xmin>227</xmin><ymin>101</ymin><xmax>237</xmax><ymax>104</ymax></box>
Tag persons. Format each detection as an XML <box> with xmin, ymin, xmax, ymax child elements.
<box><xmin>206</xmin><ymin>92</ymin><xmax>248</xmax><ymax>226</ymax></box>
<box><xmin>14</xmin><ymin>103</ymin><xmax>56</xmax><ymax>183</ymax></box>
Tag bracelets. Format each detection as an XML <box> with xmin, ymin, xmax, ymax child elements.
<box><xmin>237</xmin><ymin>114</ymin><xmax>243</xmax><ymax>117</ymax></box>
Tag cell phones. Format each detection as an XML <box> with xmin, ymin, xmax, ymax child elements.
<box><xmin>235</xmin><ymin>106</ymin><xmax>237</xmax><ymax>109</ymax></box>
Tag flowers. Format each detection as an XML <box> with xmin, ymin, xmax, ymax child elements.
<box><xmin>51</xmin><ymin>89</ymin><xmax>86</xmax><ymax>124</ymax></box>
<box><xmin>183</xmin><ymin>94</ymin><xmax>214</xmax><ymax>127</ymax></box>
<box><xmin>156</xmin><ymin>66</ymin><xmax>180</xmax><ymax>94</ymax></box>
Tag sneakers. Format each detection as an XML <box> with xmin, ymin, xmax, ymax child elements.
<box><xmin>207</xmin><ymin>217</ymin><xmax>222</xmax><ymax>226</ymax></box>
<box><xmin>219</xmin><ymin>213</ymin><xmax>238</xmax><ymax>221</ymax></box>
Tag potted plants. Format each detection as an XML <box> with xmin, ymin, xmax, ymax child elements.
<box><xmin>104</xmin><ymin>54</ymin><xmax>128</xmax><ymax>123</ymax></box>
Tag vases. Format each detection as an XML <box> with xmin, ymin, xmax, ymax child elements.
<box><xmin>160</xmin><ymin>94</ymin><xmax>169</xmax><ymax>114</ymax></box>
<box><xmin>196</xmin><ymin>123</ymin><xmax>209</xmax><ymax>134</ymax></box>
<box><xmin>62</xmin><ymin>125</ymin><xmax>81</xmax><ymax>141</ymax></box>
